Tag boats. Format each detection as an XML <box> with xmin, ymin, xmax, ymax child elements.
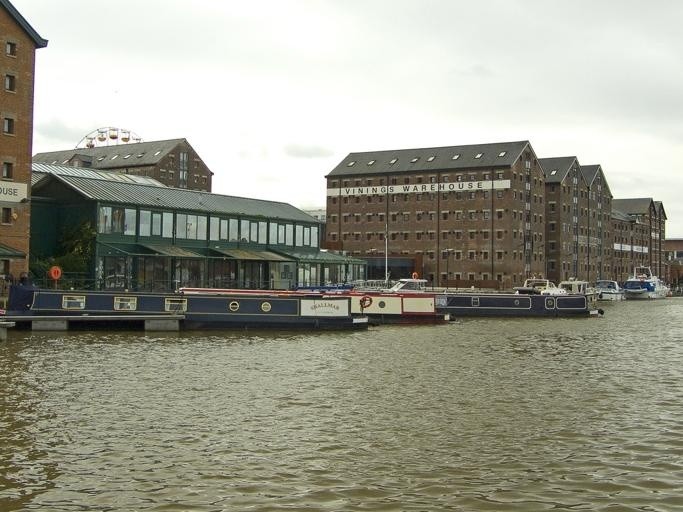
<box><xmin>508</xmin><ymin>265</ymin><xmax>672</xmax><ymax>303</ymax></box>
<box><xmin>377</xmin><ymin>277</ymin><xmax>426</xmax><ymax>294</ymax></box>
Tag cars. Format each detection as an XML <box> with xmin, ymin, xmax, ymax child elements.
<box><xmin>104</xmin><ymin>273</ymin><xmax>133</xmax><ymax>289</ymax></box>
